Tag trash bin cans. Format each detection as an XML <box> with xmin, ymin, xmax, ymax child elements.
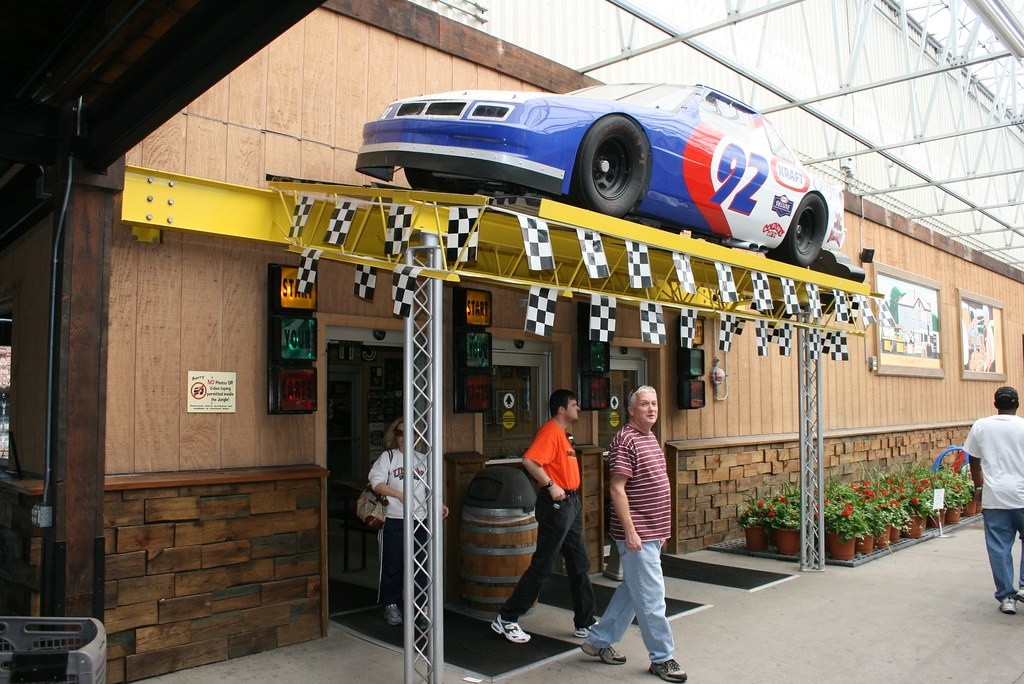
<box><xmin>456</xmin><ymin>465</ymin><xmax>539</xmax><ymax>616</ymax></box>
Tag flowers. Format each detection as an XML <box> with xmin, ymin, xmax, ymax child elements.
<box><xmin>737</xmin><ymin>458</ymin><xmax>975</xmax><ymax>545</ymax></box>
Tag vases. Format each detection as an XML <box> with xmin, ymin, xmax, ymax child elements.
<box><xmin>745</xmin><ymin>498</ymin><xmax>976</xmax><ymax>559</ymax></box>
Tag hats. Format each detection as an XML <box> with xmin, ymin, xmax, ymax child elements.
<box><xmin>995</xmin><ymin>387</ymin><xmax>1018</xmax><ymax>403</ymax></box>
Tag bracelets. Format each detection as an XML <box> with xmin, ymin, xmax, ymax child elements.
<box><xmin>975</xmin><ymin>487</ymin><xmax>982</xmax><ymax>490</ymax></box>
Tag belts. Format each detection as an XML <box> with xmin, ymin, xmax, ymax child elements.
<box><xmin>539</xmin><ymin>489</ymin><xmax>579</xmax><ymax>496</ymax></box>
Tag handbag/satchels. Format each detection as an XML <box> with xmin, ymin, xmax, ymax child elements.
<box><xmin>356</xmin><ymin>450</ymin><xmax>393</xmax><ymax>530</ymax></box>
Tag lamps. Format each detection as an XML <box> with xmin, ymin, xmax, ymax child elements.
<box><xmin>267</xmin><ymin>261</ymin><xmax>318</xmax><ymax>413</ymax></box>
<box><xmin>451</xmin><ymin>287</ymin><xmax>494</xmax><ymax>413</ymax></box>
<box><xmin>859</xmin><ymin>248</ymin><xmax>874</xmax><ymax>262</ymax></box>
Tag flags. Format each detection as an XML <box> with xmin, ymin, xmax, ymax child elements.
<box><xmin>768</xmin><ymin>276</ymin><xmax>896</xmax><ymax>362</ymax></box>
<box><xmin>518</xmin><ymin>215</ymin><xmax>666</xmax><ymax>345</ymax></box>
<box><xmin>287</xmin><ymin>194</ymin><xmax>479</xmax><ymax>317</ymax></box>
<box><xmin>673</xmin><ymin>251</ymin><xmax>773</xmax><ymax>356</ymax></box>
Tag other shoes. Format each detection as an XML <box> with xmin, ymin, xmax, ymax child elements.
<box><xmin>1015</xmin><ymin>587</ymin><xmax>1024</xmax><ymax>603</ymax></box>
<box><xmin>999</xmin><ymin>596</ymin><xmax>1017</xmax><ymax>613</ymax></box>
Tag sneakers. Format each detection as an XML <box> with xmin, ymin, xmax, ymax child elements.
<box><xmin>574</xmin><ymin>621</ymin><xmax>600</xmax><ymax>638</ymax></box>
<box><xmin>384</xmin><ymin>603</ymin><xmax>402</xmax><ymax>625</ymax></box>
<box><xmin>650</xmin><ymin>659</ymin><xmax>688</xmax><ymax>683</ymax></box>
<box><xmin>581</xmin><ymin>642</ymin><xmax>626</xmax><ymax>665</ymax></box>
<box><xmin>491</xmin><ymin>613</ymin><xmax>531</xmax><ymax>643</ymax></box>
<box><xmin>420</xmin><ymin>612</ymin><xmax>429</xmax><ymax>630</ymax></box>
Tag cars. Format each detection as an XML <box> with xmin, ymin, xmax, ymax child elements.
<box><xmin>354</xmin><ymin>80</ymin><xmax>847</xmax><ymax>270</ymax></box>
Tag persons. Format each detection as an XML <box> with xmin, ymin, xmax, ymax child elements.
<box><xmin>490</xmin><ymin>389</ymin><xmax>600</xmax><ymax>643</ymax></box>
<box><xmin>368</xmin><ymin>416</ymin><xmax>450</xmax><ymax>638</ymax></box>
<box><xmin>962</xmin><ymin>386</ymin><xmax>1024</xmax><ymax>614</ymax></box>
<box><xmin>580</xmin><ymin>385</ymin><xmax>687</xmax><ymax>682</ymax></box>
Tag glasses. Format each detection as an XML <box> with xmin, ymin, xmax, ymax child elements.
<box><xmin>394</xmin><ymin>429</ymin><xmax>417</xmax><ymax>438</ymax></box>
<box><xmin>630</xmin><ymin>385</ymin><xmax>642</xmax><ymax>400</ymax></box>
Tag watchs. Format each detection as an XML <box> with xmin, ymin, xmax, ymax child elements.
<box><xmin>545</xmin><ymin>480</ymin><xmax>553</xmax><ymax>488</ymax></box>
